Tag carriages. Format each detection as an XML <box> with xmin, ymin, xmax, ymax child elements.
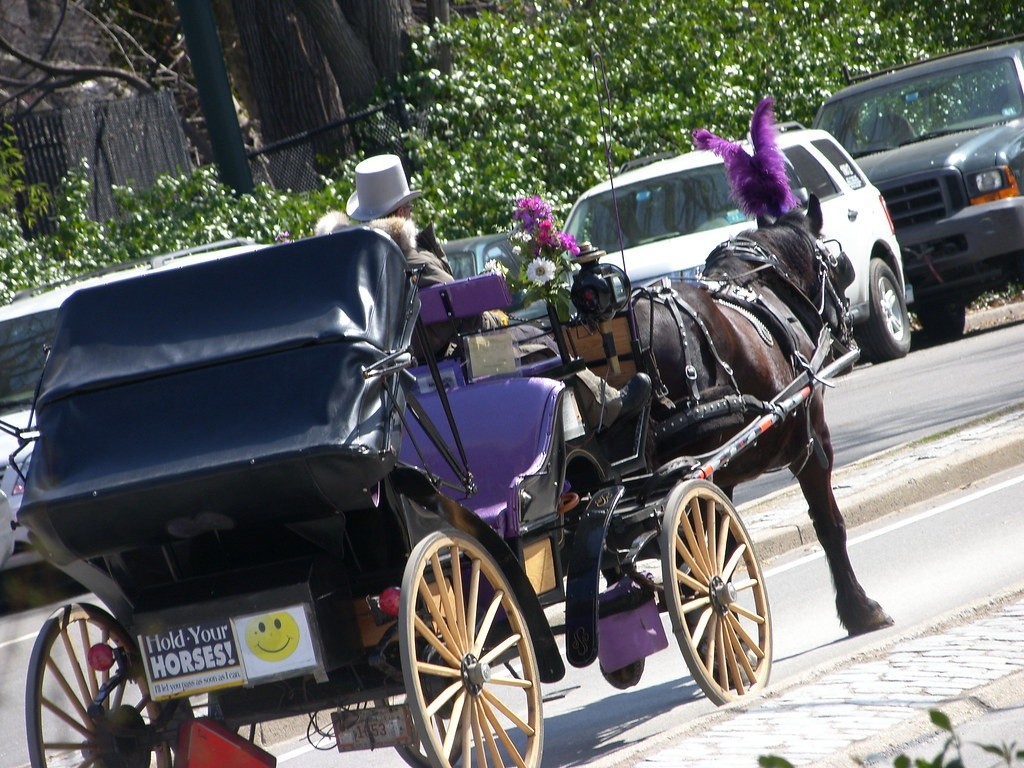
<box><xmin>17</xmin><ymin>191</ymin><xmax>893</xmax><ymax>768</ymax></box>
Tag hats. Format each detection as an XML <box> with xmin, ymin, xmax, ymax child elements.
<box><xmin>346</xmin><ymin>153</ymin><xmax>422</xmax><ymax>221</ymax></box>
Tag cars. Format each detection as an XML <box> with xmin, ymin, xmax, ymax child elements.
<box><xmin>561</xmin><ymin>121</ymin><xmax>910</xmax><ymax>363</ymax></box>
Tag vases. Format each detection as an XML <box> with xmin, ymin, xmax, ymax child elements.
<box><xmin>554</xmin><ymin>286</ymin><xmax>579</xmax><ymax>323</ymax></box>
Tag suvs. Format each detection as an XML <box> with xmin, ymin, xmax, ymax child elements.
<box><xmin>813</xmin><ymin>33</ymin><xmax>1024</xmax><ymax>337</ymax></box>
<box><xmin>1</xmin><ymin>236</ymin><xmax>253</xmax><ymax>611</ymax></box>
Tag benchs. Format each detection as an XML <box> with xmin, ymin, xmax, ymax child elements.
<box><xmin>398</xmin><ymin>374</ymin><xmax>566</xmax><ymax>542</ymax></box>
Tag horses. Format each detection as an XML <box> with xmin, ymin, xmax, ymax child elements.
<box><xmin>598</xmin><ymin>188</ymin><xmax>895</xmax><ymax>690</ymax></box>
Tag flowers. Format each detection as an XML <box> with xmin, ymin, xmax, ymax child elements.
<box><xmin>479</xmin><ymin>198</ymin><xmax>581</xmax><ymax>307</ymax></box>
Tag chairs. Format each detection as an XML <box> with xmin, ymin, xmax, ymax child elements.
<box><xmin>992</xmin><ymin>83</ymin><xmax>1011</xmax><ymax>114</ymax></box>
<box><xmin>419</xmin><ymin>273</ymin><xmax>571</xmax><ymax>386</ymax></box>
<box><xmin>870</xmin><ymin>113</ymin><xmax>918</xmax><ymax>146</ymax></box>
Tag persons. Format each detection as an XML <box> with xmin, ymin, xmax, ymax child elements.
<box><xmin>318</xmin><ymin>155</ymin><xmax>653</xmax><ymax>445</ymax></box>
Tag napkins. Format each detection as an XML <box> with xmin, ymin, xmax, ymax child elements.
<box><xmin>725</xmin><ymin>209</ymin><xmax>746</xmax><ymax>225</ymax></box>
